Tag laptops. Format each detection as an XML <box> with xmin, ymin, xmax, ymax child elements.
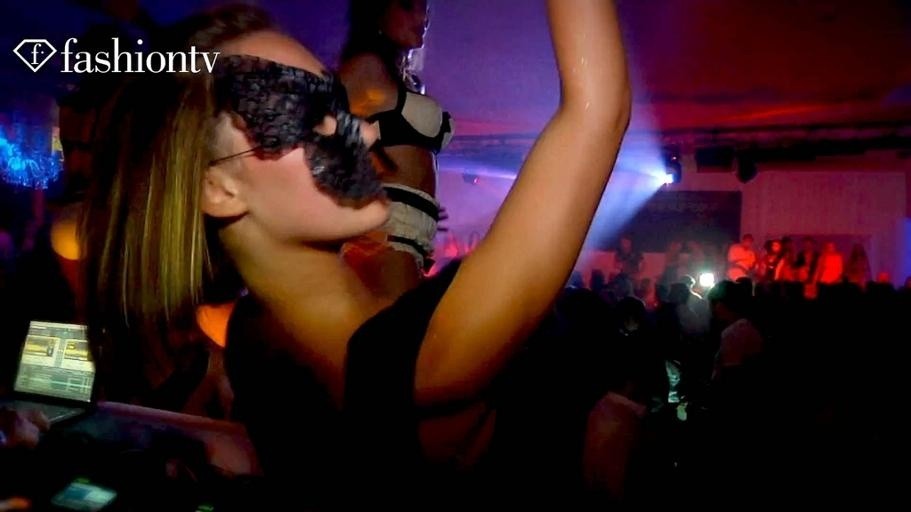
<box><xmin>0</xmin><ymin>320</ymin><xmax>97</xmax><ymax>445</ymax></box>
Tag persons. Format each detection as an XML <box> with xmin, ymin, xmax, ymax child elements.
<box><xmin>46</xmin><ymin>0</ymin><xmax>167</xmax><ymax>322</ymax></box>
<box><xmin>326</xmin><ymin>0</ymin><xmax>458</xmax><ymax>312</ymax></box>
<box><xmin>76</xmin><ymin>0</ymin><xmax>634</xmax><ymax>512</ymax></box>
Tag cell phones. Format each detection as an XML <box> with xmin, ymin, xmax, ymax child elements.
<box><xmin>48</xmin><ymin>475</ymin><xmax>117</xmax><ymax>512</ymax></box>
